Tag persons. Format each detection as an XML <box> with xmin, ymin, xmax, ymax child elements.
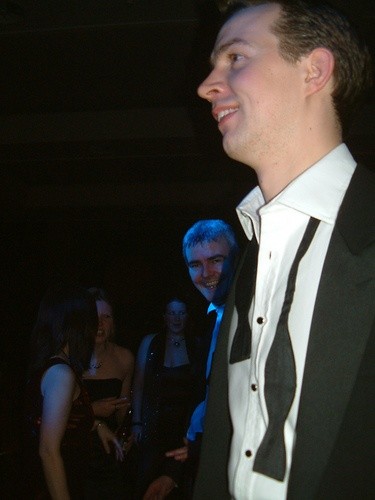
<box><xmin>20</xmin><ymin>283</ymin><xmax>207</xmax><ymax>500</ymax></box>
<box><xmin>195</xmin><ymin>0</ymin><xmax>375</xmax><ymax>500</ymax></box>
<box><xmin>182</xmin><ymin>218</ymin><xmax>241</xmax><ymax>443</ymax></box>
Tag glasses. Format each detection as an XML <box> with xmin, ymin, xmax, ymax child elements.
<box><xmin>166</xmin><ymin>313</ymin><xmax>188</xmax><ymax>322</ymax></box>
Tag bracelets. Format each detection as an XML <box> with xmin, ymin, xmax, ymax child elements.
<box><xmin>96</xmin><ymin>422</ymin><xmax>105</xmax><ymax>428</ymax></box>
